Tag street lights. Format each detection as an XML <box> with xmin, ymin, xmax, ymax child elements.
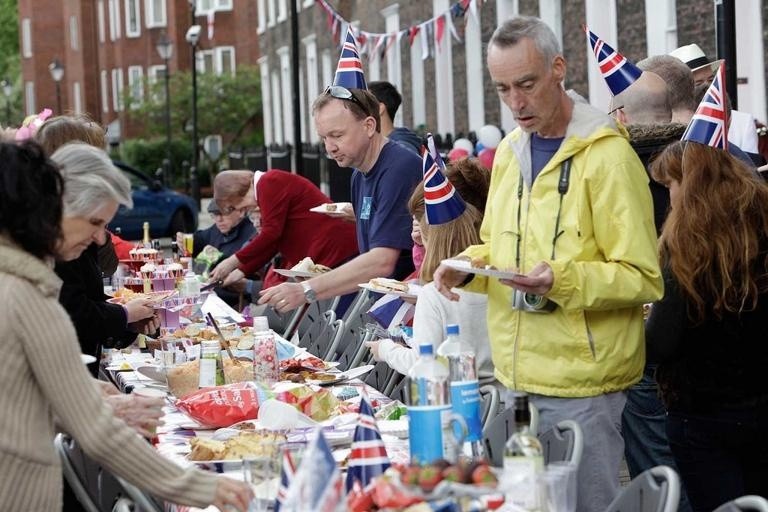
<box><xmin>153</xmin><ymin>29</ymin><xmax>174</xmax><ymax>187</ymax></box>
<box><xmin>48</xmin><ymin>57</ymin><xmax>66</xmax><ymax>117</ymax></box>
<box><xmin>1</xmin><ymin>76</ymin><xmax>13</xmax><ymax>128</ymax></box>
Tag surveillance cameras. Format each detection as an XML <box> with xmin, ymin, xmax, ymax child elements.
<box><xmin>185</xmin><ymin>25</ymin><xmax>201</xmax><ymax>44</ymax></box>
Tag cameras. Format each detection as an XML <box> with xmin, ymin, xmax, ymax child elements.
<box><xmin>512</xmin><ymin>285</ymin><xmax>554</xmax><ymax>314</ymax></box>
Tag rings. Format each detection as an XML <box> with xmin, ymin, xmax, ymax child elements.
<box><xmin>279</xmin><ymin>299</ymin><xmax>287</xmax><ymax>306</ymax></box>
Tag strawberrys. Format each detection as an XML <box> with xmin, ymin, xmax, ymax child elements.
<box><xmin>402</xmin><ymin>456</ymin><xmax>498</xmax><ymax>490</ymax></box>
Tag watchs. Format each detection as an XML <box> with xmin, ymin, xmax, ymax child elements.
<box><xmin>300</xmin><ymin>279</ymin><xmax>319</xmax><ymax>305</ymax></box>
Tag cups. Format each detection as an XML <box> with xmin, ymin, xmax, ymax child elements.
<box><xmin>179</xmin><ymin>276</ymin><xmax>200</xmax><ymax>298</ymax></box>
<box><xmin>235</xmin><ymin>432</ymin><xmax>283</xmax><ymax>511</ymax></box>
<box><xmin>252</xmin><ymin>314</ymin><xmax>271</xmax><ymax>333</ymax></box>
<box><xmin>537</xmin><ymin>460</ymin><xmax>582</xmax><ymax>512</ymax></box>
<box><xmin>253</xmin><ymin>332</ymin><xmax>280</xmax><ymax>382</ymax></box>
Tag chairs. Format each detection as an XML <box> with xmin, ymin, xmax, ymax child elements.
<box><xmin>55</xmin><ymin>272</ymin><xmax>583</xmax><ymax>512</ymax></box>
<box><xmin>717</xmin><ymin>495</ymin><xmax>767</xmax><ymax>511</ymax></box>
<box><xmin>605</xmin><ymin>466</ymin><xmax>679</xmax><ymax>512</ymax></box>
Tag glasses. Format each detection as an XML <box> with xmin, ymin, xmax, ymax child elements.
<box><xmin>323</xmin><ymin>84</ymin><xmax>370</xmax><ymax>117</ymax></box>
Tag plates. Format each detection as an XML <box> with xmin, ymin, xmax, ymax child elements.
<box><xmin>289</xmin><ymin>359</ymin><xmax>375</xmax><ymax>386</ymax></box>
<box><xmin>106</xmin><ymin>289</ymin><xmax>173</xmax><ymax>307</ymax></box>
<box><xmin>158</xmin><ymin>413</ymin><xmax>408</xmax><ymax>464</ymax></box>
<box><xmin>78</xmin><ymin>352</ymin><xmax>98</xmax><ymax>365</ymax></box>
<box><xmin>103</xmin><ymin>359</ymin><xmax>214</xmax><ymax>431</ymax></box>
<box><xmin>273</xmin><ymin>184</ymin><xmax>521</xmax><ymax>297</ymax></box>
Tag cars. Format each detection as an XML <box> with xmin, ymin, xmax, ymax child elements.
<box><xmin>104</xmin><ymin>161</ymin><xmax>199</xmax><ymax>242</ymax></box>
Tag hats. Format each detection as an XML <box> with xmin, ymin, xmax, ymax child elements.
<box><xmin>420</xmin><ymin>144</ymin><xmax>468</xmax><ymax>226</ymax></box>
<box><xmin>581</xmin><ymin>23</ymin><xmax>644</xmax><ymax>97</ymax></box>
<box><xmin>679</xmin><ymin>59</ymin><xmax>730</xmax><ymax>154</ymax></box>
<box><xmin>668</xmin><ymin>43</ymin><xmax>722</xmax><ymax>73</ymax></box>
<box><xmin>207</xmin><ymin>199</ymin><xmax>235</xmax><ymax>213</ymax></box>
<box><xmin>332</xmin><ymin>24</ymin><xmax>369</xmax><ymax>92</ymax></box>
<box><xmin>426</xmin><ymin>132</ymin><xmax>448</xmax><ymax>172</ymax></box>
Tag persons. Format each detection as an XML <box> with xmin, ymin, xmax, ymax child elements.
<box><xmin>187</xmin><ymin>198</ymin><xmax>258</xmax><ymax>306</ymax></box>
<box><xmin>646</xmin><ymin>142</ymin><xmax>766</xmax><ymax>511</ymax></box>
<box><xmin>600</xmin><ymin>72</ymin><xmax>692</xmax><ymax>511</ymax></box>
<box><xmin>259</xmin><ymin>85</ymin><xmax>426</xmax><ymax>315</ymax></box>
<box><xmin>47</xmin><ymin>141</ymin><xmax>135</xmax><ymax>264</ymax></box>
<box><xmin>209</xmin><ymin>165</ymin><xmax>359</xmax><ymax>321</ymax></box>
<box><xmin>27</xmin><ymin>110</ymin><xmax>163</xmax><ymax>381</ymax></box>
<box><xmin>0</xmin><ymin>137</ymin><xmax>255</xmax><ymax>511</ymax></box>
<box><xmin>433</xmin><ymin>15</ymin><xmax>667</xmax><ymax>511</ymax></box>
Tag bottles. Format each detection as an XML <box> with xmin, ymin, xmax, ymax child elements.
<box><xmin>198</xmin><ymin>339</ymin><xmax>224</xmax><ymax>387</ymax></box>
<box><xmin>504</xmin><ymin>390</ymin><xmax>544</xmax><ymax>511</ymax></box>
<box><xmin>140</xmin><ymin>220</ymin><xmax>153</xmax><ymax>249</ymax></box>
<box><xmin>408</xmin><ymin>322</ymin><xmax>484</xmax><ymax>471</ymax></box>
<box><xmin>179</xmin><ymin>237</ymin><xmax>194</xmax><ymax>272</ymax></box>
<box><xmin>114</xmin><ymin>226</ymin><xmax>123</xmax><ymax>239</ymax></box>
